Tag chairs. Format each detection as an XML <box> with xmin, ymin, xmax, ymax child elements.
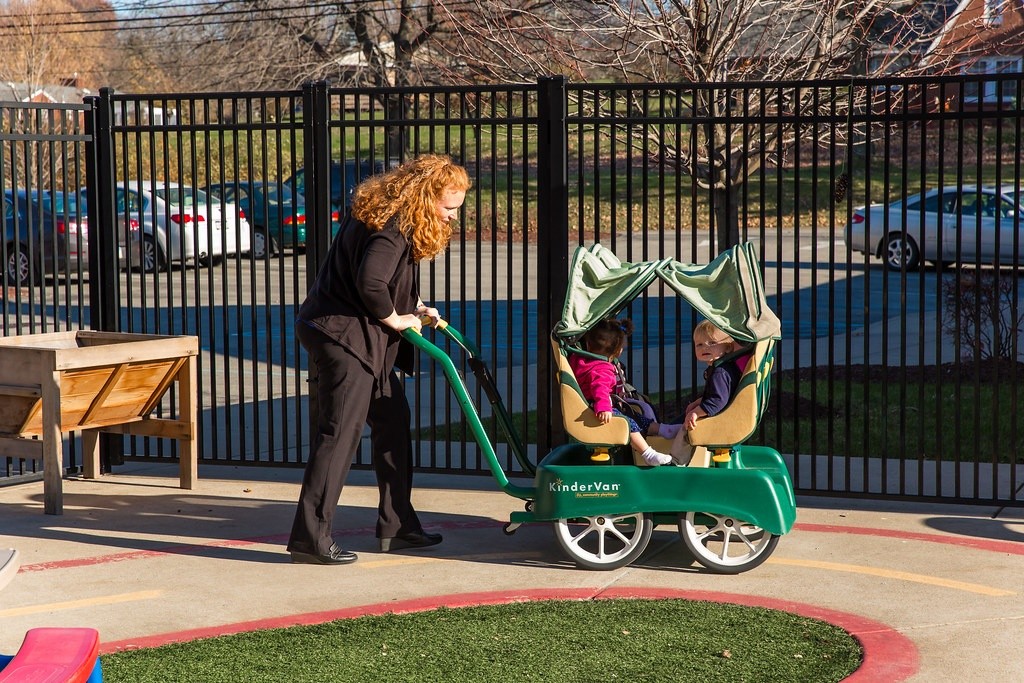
<box><xmin>551</xmin><ymin>318</ymin><xmax>645</xmax><ymax>465</ymax></box>
<box><xmin>688</xmin><ymin>337</ymin><xmax>776</xmax><ymax>468</ymax></box>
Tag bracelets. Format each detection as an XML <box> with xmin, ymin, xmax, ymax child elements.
<box><xmin>414</xmin><ymin>303</ymin><xmax>425</xmax><ymax>311</ymax></box>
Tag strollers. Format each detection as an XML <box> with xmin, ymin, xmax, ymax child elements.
<box><xmin>398</xmin><ymin>242</ymin><xmax>797</xmax><ymax>574</ymax></box>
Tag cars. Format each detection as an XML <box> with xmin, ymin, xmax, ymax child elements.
<box><xmin>80</xmin><ymin>181</ymin><xmax>250</xmax><ymax>273</ymax></box>
<box><xmin>5</xmin><ymin>190</ymin><xmax>141</xmax><ymax>286</ymax></box>
<box><xmin>844</xmin><ymin>186</ymin><xmax>1024</xmax><ymax>272</ymax></box>
<box><xmin>200</xmin><ymin>181</ymin><xmax>343</xmax><ymax>260</ymax></box>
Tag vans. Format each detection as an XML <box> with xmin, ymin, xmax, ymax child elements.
<box><xmin>283</xmin><ymin>161</ymin><xmax>385</xmax><ymax>214</ymax></box>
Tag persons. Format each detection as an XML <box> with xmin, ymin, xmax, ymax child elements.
<box><xmin>285</xmin><ymin>147</ymin><xmax>471</xmax><ymax>568</ymax></box>
<box><xmin>661</xmin><ymin>318</ymin><xmax>744</xmax><ymax>467</ymax></box>
<box><xmin>568</xmin><ymin>315</ymin><xmax>686</xmax><ymax>467</ymax></box>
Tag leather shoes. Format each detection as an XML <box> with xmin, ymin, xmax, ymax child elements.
<box><xmin>291</xmin><ymin>541</ymin><xmax>358</xmax><ymax>564</ymax></box>
<box><xmin>380</xmin><ymin>527</ymin><xmax>443</xmax><ymax>552</ymax></box>
